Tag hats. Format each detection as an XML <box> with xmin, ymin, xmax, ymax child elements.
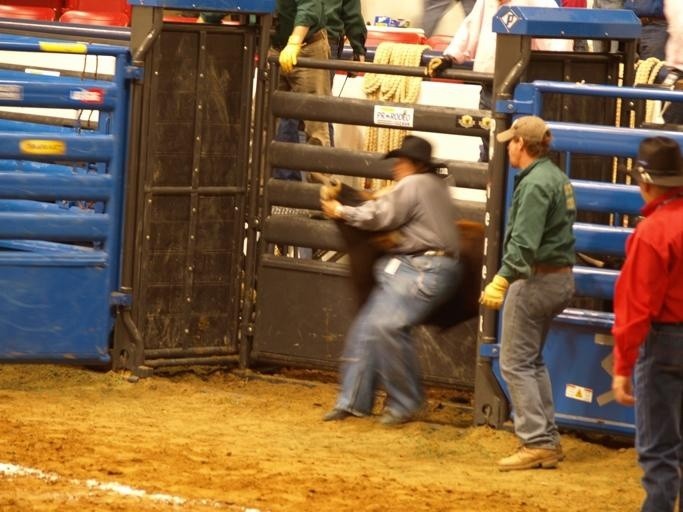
<box><xmin>618</xmin><ymin>137</ymin><xmax>682</xmax><ymax>187</ymax></box>
<box><xmin>379</xmin><ymin>136</ymin><xmax>447</xmax><ymax>169</ymax></box>
<box><xmin>496</xmin><ymin>116</ymin><xmax>551</xmax><ymax>143</ymax></box>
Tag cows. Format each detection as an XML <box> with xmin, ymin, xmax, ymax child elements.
<box><xmin>320</xmin><ymin>178</ymin><xmax>605</xmax><ymax>416</ymax></box>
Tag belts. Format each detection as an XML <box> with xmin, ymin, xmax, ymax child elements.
<box><xmin>640</xmin><ymin>15</ymin><xmax>666</xmax><ymax>25</ymax></box>
<box><xmin>273</xmin><ymin>28</ymin><xmax>327</xmax><ymax>52</ymax></box>
<box><xmin>532</xmin><ymin>265</ymin><xmax>573</xmax><ymax>273</ymax></box>
<box><xmin>412</xmin><ymin>249</ymin><xmax>460</xmax><ymax>257</ymax></box>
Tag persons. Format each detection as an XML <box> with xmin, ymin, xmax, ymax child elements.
<box><xmin>197</xmin><ymin>1</ymin><xmax>683</xmax><ymax>262</ymax></box>
<box><xmin>479</xmin><ymin>113</ymin><xmax>577</xmax><ymax>469</ymax></box>
<box><xmin>313</xmin><ymin>135</ymin><xmax>460</xmax><ymax>426</ymax></box>
<box><xmin>612</xmin><ymin>137</ymin><xmax>683</xmax><ymax>512</ymax></box>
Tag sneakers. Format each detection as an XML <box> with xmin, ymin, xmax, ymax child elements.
<box><xmin>376</xmin><ymin>414</ymin><xmax>402</xmax><ymax>426</ymax></box>
<box><xmin>497</xmin><ymin>438</ymin><xmax>565</xmax><ymax>471</ymax></box>
<box><xmin>322</xmin><ymin>410</ymin><xmax>350</xmax><ymax>422</ymax></box>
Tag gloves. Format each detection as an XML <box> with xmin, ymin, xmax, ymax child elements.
<box><xmin>348</xmin><ymin>53</ymin><xmax>366</xmax><ymax>77</ymax></box>
<box><xmin>424</xmin><ymin>55</ymin><xmax>454</xmax><ymax>77</ymax></box>
<box><xmin>278</xmin><ymin>35</ymin><xmax>304</xmax><ymax>73</ymax></box>
<box><xmin>479</xmin><ymin>274</ymin><xmax>509</xmax><ymax>310</ymax></box>
